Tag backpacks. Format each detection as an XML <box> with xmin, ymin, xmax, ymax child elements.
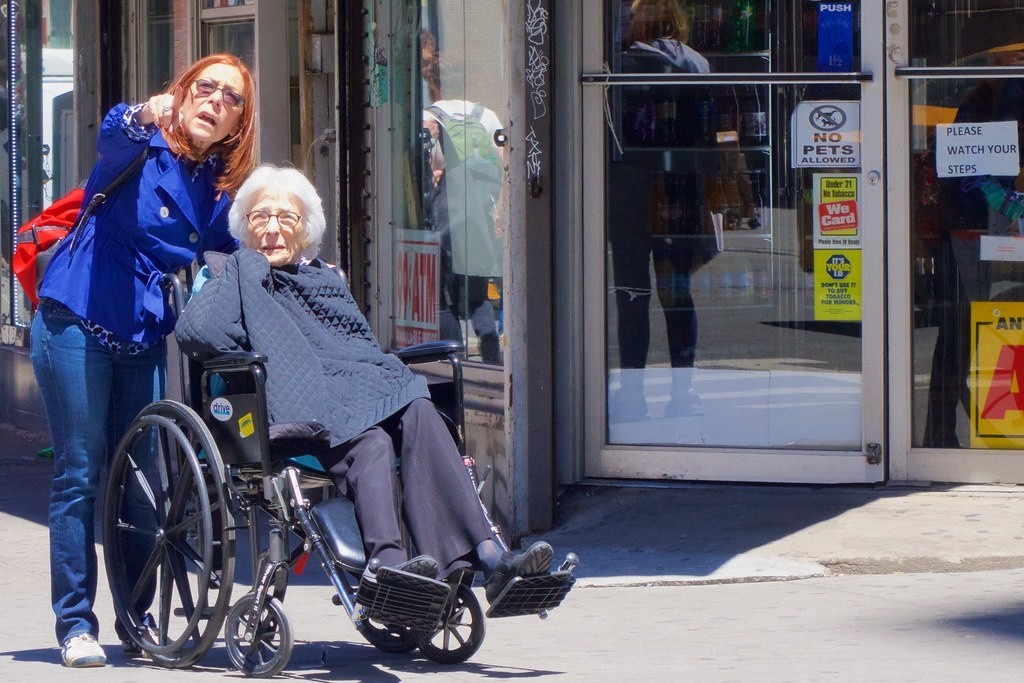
<box><xmin>12</xmin><ymin>146</ymin><xmax>149</xmax><ymax>311</ymax></box>
<box><xmin>426</xmin><ymin>104</ymin><xmax>502</xmax><ymax>219</ymax></box>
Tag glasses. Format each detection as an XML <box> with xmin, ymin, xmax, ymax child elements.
<box><xmin>246</xmin><ymin>211</ymin><xmax>301</xmax><ymax>228</ymax></box>
<box><xmin>193</xmin><ymin>79</ymin><xmax>244</xmax><ymax>107</ymax></box>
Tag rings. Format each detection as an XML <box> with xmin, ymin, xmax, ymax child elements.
<box><xmin>163</xmin><ymin>106</ymin><xmax>171</xmax><ymax>112</ymax></box>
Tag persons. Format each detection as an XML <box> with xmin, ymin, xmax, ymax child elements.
<box><xmin>32</xmin><ymin>52</ymin><xmax>262</xmax><ymax>667</ymax></box>
<box><xmin>605</xmin><ymin>0</ymin><xmax>770</xmax><ymax>425</ymax></box>
<box><xmin>174</xmin><ymin>161</ymin><xmax>556</xmax><ymax>622</ymax></box>
<box><xmin>410</xmin><ymin>32</ymin><xmax>505</xmax><ymax>365</ymax></box>
<box><xmin>921</xmin><ymin>50</ymin><xmax>1024</xmax><ymax>448</ymax></box>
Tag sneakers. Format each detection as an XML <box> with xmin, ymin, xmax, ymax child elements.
<box><xmin>61</xmin><ymin>633</ymin><xmax>106</xmax><ymax>667</ymax></box>
<box><xmin>122</xmin><ymin>627</ymin><xmax>184</xmax><ymax>659</ymax></box>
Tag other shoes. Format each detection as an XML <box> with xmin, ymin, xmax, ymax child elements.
<box><xmin>610</xmin><ymin>389</ymin><xmax>649</xmax><ymax>422</ymax></box>
<box><xmin>482</xmin><ymin>541</ymin><xmax>554</xmax><ymax>606</ymax></box>
<box><xmin>665</xmin><ymin>388</ymin><xmax>703</xmax><ymax>416</ymax></box>
<box><xmin>391</xmin><ymin>555</ymin><xmax>440</xmax><ymax>580</ymax></box>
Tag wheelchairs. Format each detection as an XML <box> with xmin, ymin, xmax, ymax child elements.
<box><xmin>100</xmin><ymin>274</ymin><xmax>580</xmax><ymax>679</ymax></box>
